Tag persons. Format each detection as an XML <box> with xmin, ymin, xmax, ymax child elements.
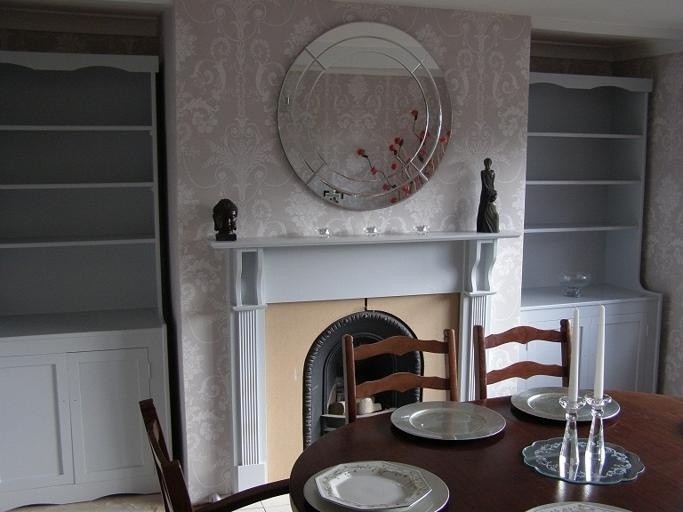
<box><xmin>476</xmin><ymin>157</ymin><xmax>500</xmax><ymax>233</ymax></box>
<box><xmin>212</xmin><ymin>198</ymin><xmax>238</xmax><ymax>234</ymax></box>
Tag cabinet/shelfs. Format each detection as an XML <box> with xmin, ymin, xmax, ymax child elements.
<box><xmin>203</xmin><ymin>229</ymin><xmax>521</xmax><ymax>496</ymax></box>
<box><xmin>520</xmin><ymin>69</ymin><xmax>664</xmax><ymax>396</ymax></box>
<box><xmin>0</xmin><ymin>50</ymin><xmax>173</xmax><ymax>512</ymax></box>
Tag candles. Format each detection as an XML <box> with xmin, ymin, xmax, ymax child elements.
<box><xmin>594</xmin><ymin>306</ymin><xmax>606</xmax><ymax>398</ymax></box>
<box><xmin>569</xmin><ymin>307</ymin><xmax>580</xmax><ymax>405</ymax></box>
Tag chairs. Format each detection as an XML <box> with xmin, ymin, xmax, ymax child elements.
<box><xmin>135</xmin><ymin>396</ymin><xmax>290</xmax><ymax>511</ymax></box>
<box><xmin>336</xmin><ymin>318</ymin><xmax>571</xmax><ymax>426</ymax></box>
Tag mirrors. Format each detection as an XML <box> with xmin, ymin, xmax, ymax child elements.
<box><xmin>276</xmin><ymin>23</ymin><xmax>453</xmax><ymax>213</ymax></box>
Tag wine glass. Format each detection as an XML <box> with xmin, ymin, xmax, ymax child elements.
<box><xmin>559</xmin><ymin>270</ymin><xmax>593</xmax><ymax>298</ymax></box>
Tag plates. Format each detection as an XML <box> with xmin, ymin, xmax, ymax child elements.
<box><xmin>390</xmin><ymin>399</ymin><xmax>506</xmax><ymax>443</ymax></box>
<box><xmin>303</xmin><ymin>462</ymin><xmax>450</xmax><ymax>511</ymax></box>
<box><xmin>524</xmin><ymin>501</ymin><xmax>635</xmax><ymax>512</ymax></box>
<box><xmin>314</xmin><ymin>461</ymin><xmax>432</xmax><ymax>509</ymax></box>
<box><xmin>510</xmin><ymin>386</ymin><xmax>620</xmax><ymax>423</ymax></box>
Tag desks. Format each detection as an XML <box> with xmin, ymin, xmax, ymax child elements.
<box><xmin>287</xmin><ymin>389</ymin><xmax>683</xmax><ymax>511</ymax></box>
